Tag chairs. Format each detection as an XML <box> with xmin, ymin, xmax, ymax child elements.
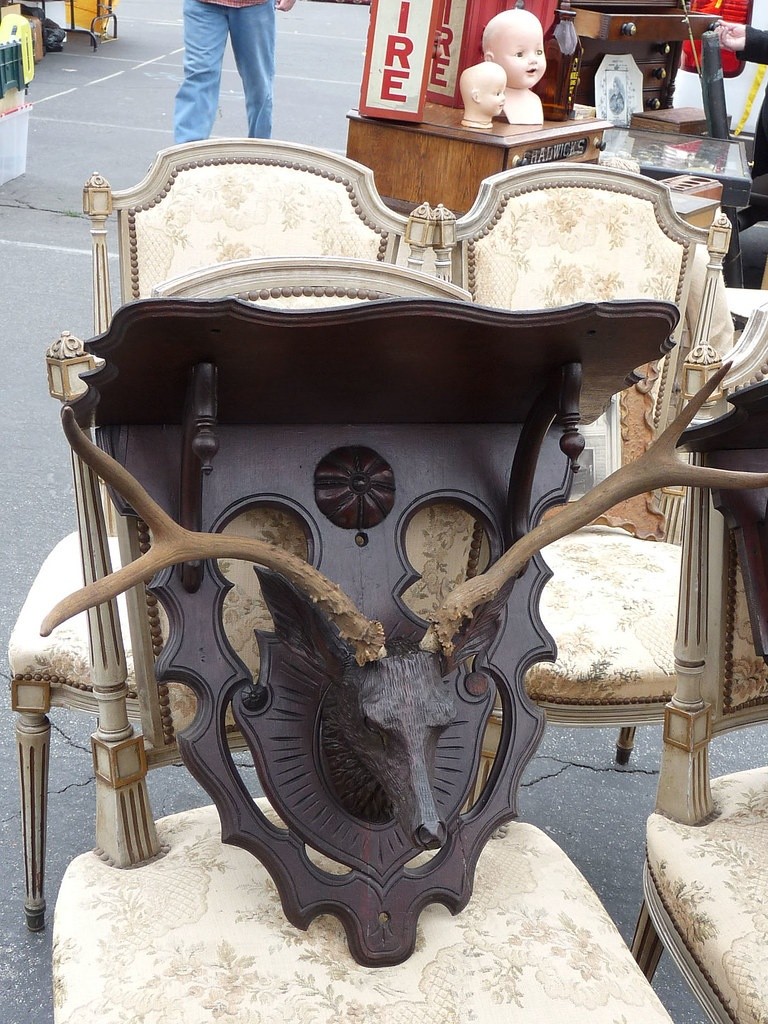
<box><xmin>630</xmin><ymin>294</ymin><xmax>768</xmax><ymax>1024</ymax></box>
<box><xmin>4</xmin><ymin>136</ymin><xmax>431</xmax><ymax>929</ymax></box>
<box><xmin>49</xmin><ymin>299</ymin><xmax>683</xmax><ymax>1024</ymax></box>
<box><xmin>429</xmin><ymin>156</ymin><xmax>734</xmax><ymax>815</ymax></box>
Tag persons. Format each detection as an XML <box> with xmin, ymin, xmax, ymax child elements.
<box><xmin>483</xmin><ymin>9</ymin><xmax>549</xmax><ymax>125</ymax></box>
<box><xmin>462</xmin><ymin>61</ymin><xmax>507</xmax><ymax>128</ymax></box>
<box><xmin>172</xmin><ymin>0</ymin><xmax>297</xmax><ymax>145</ymax></box>
<box><xmin>707</xmin><ymin>19</ymin><xmax>768</xmax><ymax>331</ymax></box>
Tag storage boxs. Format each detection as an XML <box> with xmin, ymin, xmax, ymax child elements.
<box><xmin>0</xmin><ymin>106</ymin><xmax>34</xmax><ymax>184</ymax></box>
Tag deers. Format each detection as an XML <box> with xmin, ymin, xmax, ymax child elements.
<box><xmin>38</xmin><ymin>362</ymin><xmax>768</xmax><ymax>852</ymax></box>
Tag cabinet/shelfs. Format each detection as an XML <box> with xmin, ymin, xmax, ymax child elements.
<box><xmin>347</xmin><ymin>102</ymin><xmax>614</xmax><ymax>223</ymax></box>
<box><xmin>600</xmin><ymin>126</ymin><xmax>752</xmax><ymax>290</ymax></box>
<box><xmin>628</xmin><ymin>106</ymin><xmax>732</xmax><ymax>136</ymax></box>
<box><xmin>534</xmin><ymin>0</ymin><xmax>724</xmax><ymax>121</ymax></box>
